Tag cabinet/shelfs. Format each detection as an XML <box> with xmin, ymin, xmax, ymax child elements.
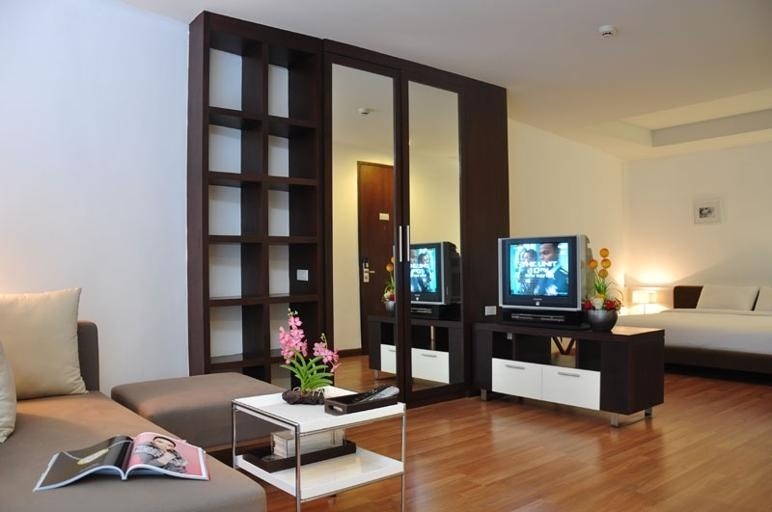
<box><xmin>323</xmin><ymin>37</ymin><xmax>510</xmax><ymax>409</ymax></box>
<box><xmin>188</xmin><ymin>10</ymin><xmax>328</xmax><ymax>384</ymax></box>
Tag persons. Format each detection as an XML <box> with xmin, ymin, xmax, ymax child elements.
<box><xmin>533</xmin><ymin>243</ymin><xmax>569</xmax><ymax>296</ymax></box>
<box><xmin>410</xmin><ymin>249</ymin><xmax>420</xmax><ymax>293</ymax></box>
<box><xmin>417</xmin><ymin>254</ymin><xmax>432</xmax><ymax>291</ymax></box>
<box><xmin>517</xmin><ymin>249</ymin><xmax>538</xmax><ymax>294</ymax></box>
<box><xmin>135</xmin><ymin>435</ymin><xmax>187</xmax><ymax>473</ymax></box>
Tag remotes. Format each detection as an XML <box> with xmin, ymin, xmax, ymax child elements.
<box><xmin>344</xmin><ymin>384</ymin><xmax>400</xmax><ymax>404</ymax></box>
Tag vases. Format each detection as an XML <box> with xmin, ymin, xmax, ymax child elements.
<box><xmin>386</xmin><ymin>301</ymin><xmax>396</xmax><ymax>315</ymax></box>
<box><xmin>587</xmin><ymin>310</ymin><xmax>617</xmax><ymax>332</ymax></box>
<box><xmin>282</xmin><ymin>390</ymin><xmax>325</xmax><ymax>404</ymax></box>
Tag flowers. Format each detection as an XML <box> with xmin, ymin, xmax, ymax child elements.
<box><xmin>582</xmin><ymin>247</ymin><xmax>624</xmax><ymax>311</ymax></box>
<box><xmin>380</xmin><ymin>256</ymin><xmax>396</xmax><ymax>305</ymax></box>
<box><xmin>279</xmin><ymin>309</ymin><xmax>343</xmax><ymax>395</ymax></box>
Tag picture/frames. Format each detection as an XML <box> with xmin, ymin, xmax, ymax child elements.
<box><xmin>693</xmin><ymin>201</ymin><xmax>718</xmax><ymax>223</ymax></box>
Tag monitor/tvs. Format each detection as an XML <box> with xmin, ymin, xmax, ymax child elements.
<box><xmin>497</xmin><ymin>233</ymin><xmax>586</xmax><ymax>325</ymax></box>
<box><xmin>408</xmin><ymin>240</ymin><xmax>461</xmax><ymax>318</ymax></box>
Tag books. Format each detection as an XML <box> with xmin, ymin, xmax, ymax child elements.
<box><xmin>31</xmin><ymin>432</ymin><xmax>209</xmax><ymax>493</ymax></box>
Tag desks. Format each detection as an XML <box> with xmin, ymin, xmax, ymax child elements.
<box><xmin>473</xmin><ymin>321</ymin><xmax>665</xmax><ymax>427</ymax></box>
<box><xmin>366</xmin><ymin>315</ymin><xmax>462</xmax><ymax>384</ymax></box>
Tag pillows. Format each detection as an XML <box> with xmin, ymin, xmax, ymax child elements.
<box><xmin>754</xmin><ymin>287</ymin><xmax>772</xmax><ymax>311</ymax></box>
<box><xmin>696</xmin><ymin>286</ymin><xmax>760</xmax><ymax>310</ymax></box>
<box><xmin>0</xmin><ymin>288</ymin><xmax>87</xmax><ymax>399</ymax></box>
<box><xmin>0</xmin><ymin>338</ymin><xmax>17</xmax><ymax>443</ymax></box>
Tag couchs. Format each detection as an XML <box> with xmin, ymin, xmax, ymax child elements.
<box><xmin>112</xmin><ymin>371</ymin><xmax>283</xmax><ymax>455</ymax></box>
<box><xmin>0</xmin><ymin>322</ymin><xmax>267</xmax><ymax>512</ymax></box>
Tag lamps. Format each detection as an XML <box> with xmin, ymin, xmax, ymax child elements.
<box><xmin>632</xmin><ymin>290</ymin><xmax>658</xmax><ymax>316</ymax></box>
